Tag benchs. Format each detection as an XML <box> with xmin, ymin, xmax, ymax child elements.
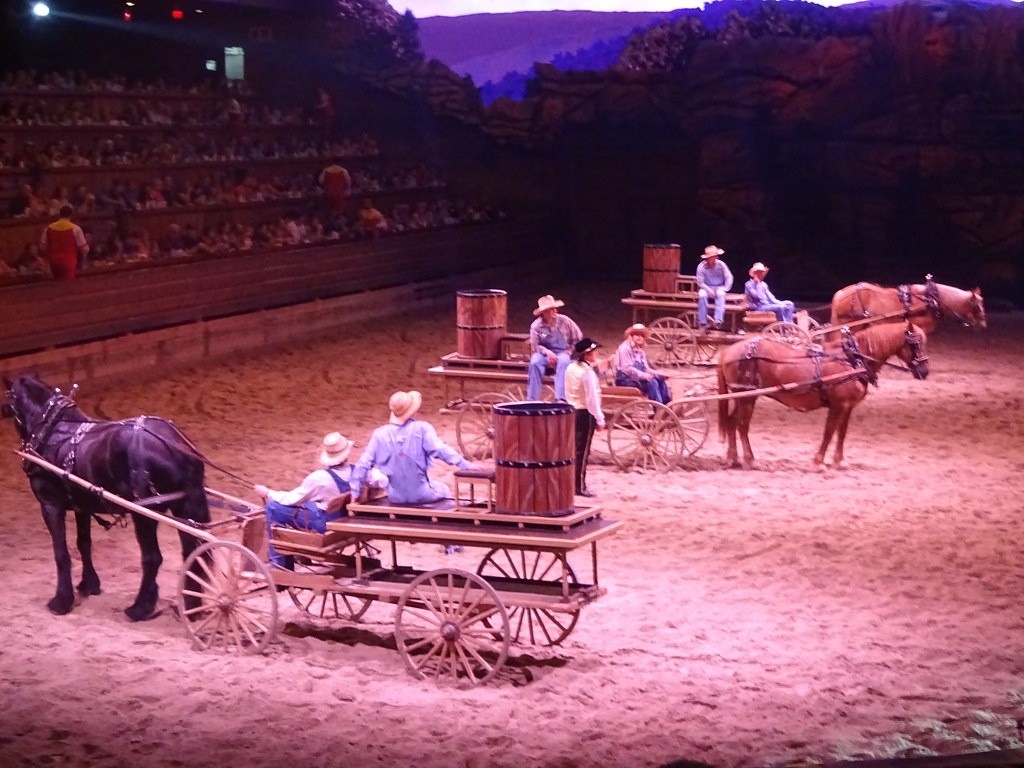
<box><xmin>591</xmin><ymin>353</ymin><xmax>654</xmax><ymax>414</ymax></box>
<box><xmin>745</xmin><ymin>308</ymin><xmax>780</xmax><ymax>328</ymax></box>
<box><xmin>269</xmin><ymin>481</ymin><xmax>389</xmax><ymax>559</ymax></box>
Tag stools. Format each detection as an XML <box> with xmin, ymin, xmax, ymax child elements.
<box><xmin>677</xmin><ymin>274</ymin><xmax>698</xmax><ymax>294</ymax></box>
<box><xmin>453</xmin><ymin>468</ymin><xmax>496</xmax><ymax>513</ymax></box>
<box><xmin>500</xmin><ymin>332</ymin><xmax>533</xmax><ymax>363</ymax></box>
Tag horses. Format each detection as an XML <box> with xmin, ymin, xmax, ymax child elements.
<box><xmin>714</xmin><ymin>318</ymin><xmax>929</xmax><ymax>471</ymax></box>
<box><xmin>2</xmin><ymin>368</ymin><xmax>211</xmax><ymax>625</ymax></box>
<box><xmin>831</xmin><ymin>281</ymin><xmax>988</xmax><ymax>341</ymax></box>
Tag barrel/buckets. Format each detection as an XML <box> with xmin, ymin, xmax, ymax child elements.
<box><xmin>457</xmin><ymin>288</ymin><xmax>509</xmax><ymax>358</ymax></box>
<box><xmin>644</xmin><ymin>243</ymin><xmax>681</xmax><ymax>293</ymax></box>
<box><xmin>492</xmin><ymin>400</ymin><xmax>575</xmax><ymax>516</ymax></box>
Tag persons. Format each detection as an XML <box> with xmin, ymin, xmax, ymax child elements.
<box><xmin>745</xmin><ymin>263</ymin><xmax>796</xmax><ymax>333</ymax></box>
<box><xmin>39</xmin><ymin>205</ymin><xmax>90</xmax><ymax>279</ymax></box>
<box><xmin>255</xmin><ymin>431</ymin><xmax>388</xmax><ymax>571</ymax></box>
<box><xmin>564</xmin><ymin>337</ymin><xmax>605</xmax><ymax>497</ymax></box>
<box><xmin>696</xmin><ymin>246</ymin><xmax>733</xmax><ymax>329</ymax></box>
<box><xmin>526</xmin><ymin>295</ymin><xmax>582</xmax><ymax>400</ymax></box>
<box><xmin>0</xmin><ymin>66</ymin><xmax>505</xmax><ymax>282</ymax></box>
<box><xmin>350</xmin><ymin>391</ymin><xmax>479</xmax><ymax>554</ymax></box>
<box><xmin>614</xmin><ymin>324</ymin><xmax>676</xmax><ymax>417</ymax></box>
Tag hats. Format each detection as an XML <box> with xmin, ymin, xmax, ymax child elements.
<box><xmin>319</xmin><ymin>432</ymin><xmax>354</xmax><ymax>467</ymax></box>
<box><xmin>748</xmin><ymin>262</ymin><xmax>769</xmax><ymax>278</ymax></box>
<box><xmin>570</xmin><ymin>338</ymin><xmax>602</xmax><ymax>360</ymax></box>
<box><xmin>701</xmin><ymin>245</ymin><xmax>724</xmax><ymax>259</ymax></box>
<box><xmin>389</xmin><ymin>391</ymin><xmax>422</xmax><ymax>425</ymax></box>
<box><xmin>533</xmin><ymin>295</ymin><xmax>566</xmax><ymax>315</ymax></box>
<box><xmin>624</xmin><ymin>323</ymin><xmax>650</xmax><ymax>339</ymax></box>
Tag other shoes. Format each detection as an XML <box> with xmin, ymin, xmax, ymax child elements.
<box><xmin>580</xmin><ymin>490</ymin><xmax>596</xmax><ymax>497</ymax></box>
<box><xmin>698</xmin><ymin>325</ymin><xmax>709</xmax><ymax>334</ymax></box>
<box><xmin>713</xmin><ymin>320</ymin><xmax>722</xmax><ymax>329</ymax></box>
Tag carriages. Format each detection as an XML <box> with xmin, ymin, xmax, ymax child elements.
<box><xmin>621</xmin><ymin>274</ymin><xmax>989</xmax><ymax>374</ymax></box>
<box><xmin>426</xmin><ymin>318</ymin><xmax>932</xmax><ymax>477</ymax></box>
<box><xmin>0</xmin><ymin>371</ymin><xmax>626</xmax><ymax>687</ymax></box>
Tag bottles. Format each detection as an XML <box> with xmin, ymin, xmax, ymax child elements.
<box><xmin>77</xmin><ymin>250</ymin><xmax>87</xmax><ymax>269</ymax></box>
